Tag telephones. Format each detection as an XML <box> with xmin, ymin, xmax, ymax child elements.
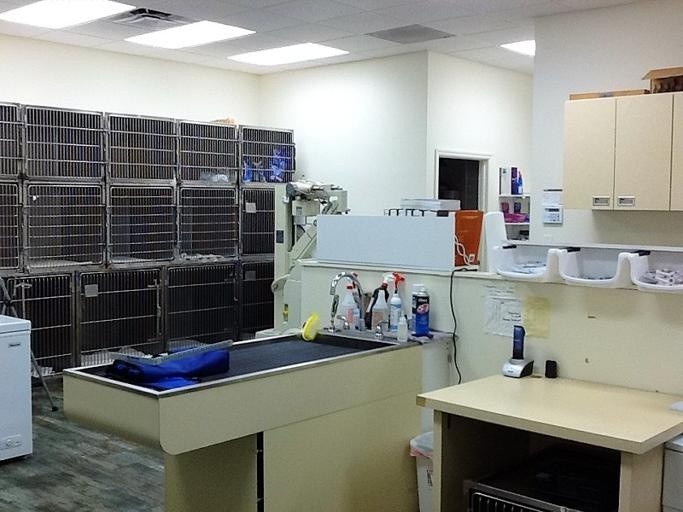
<box><xmin>503</xmin><ymin>324</ymin><xmax>534</xmax><ymax>378</ymax></box>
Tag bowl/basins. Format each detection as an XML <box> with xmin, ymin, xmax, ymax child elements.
<box><xmin>505</xmin><ymin>213</ymin><xmax>527</xmax><ymax>223</ymax></box>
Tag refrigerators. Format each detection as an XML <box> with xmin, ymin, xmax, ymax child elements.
<box><xmin>0</xmin><ymin>313</ymin><xmax>33</xmax><ymax>464</ymax></box>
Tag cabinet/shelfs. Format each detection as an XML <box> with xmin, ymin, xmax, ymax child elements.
<box><xmin>498</xmin><ymin>195</ymin><xmax>530</xmax><ymax>242</ymax></box>
<box><xmin>563</xmin><ymin>92</ymin><xmax>683</xmax><ymax>212</ymax></box>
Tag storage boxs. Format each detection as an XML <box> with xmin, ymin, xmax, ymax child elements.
<box><xmin>641</xmin><ymin>67</ymin><xmax>683</xmax><ymax>93</ymax></box>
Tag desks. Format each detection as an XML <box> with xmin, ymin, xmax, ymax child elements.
<box><xmin>416</xmin><ymin>370</ymin><xmax>683</xmax><ymax>512</ymax></box>
<box><xmin>62</xmin><ymin>331</ymin><xmax>453</xmax><ymax>512</ymax></box>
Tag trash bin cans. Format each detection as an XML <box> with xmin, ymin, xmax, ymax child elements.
<box><xmin>409</xmin><ymin>430</ymin><xmax>432</xmax><ymax>512</ymax></box>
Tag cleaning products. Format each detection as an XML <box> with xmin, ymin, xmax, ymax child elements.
<box><xmin>365</xmin><ymin>272</ymin><xmax>402</xmax><ymax>330</ymax></box>
<box><xmin>514</xmin><ymin>171</ymin><xmax>523</xmax><ymax>194</ymax></box>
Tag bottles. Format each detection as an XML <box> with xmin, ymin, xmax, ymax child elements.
<box><xmin>342</xmin><ymin>284</ymin><xmax>430</xmax><ymax>342</ymax></box>
<box><xmin>511</xmin><ymin>167</ymin><xmax>523</xmax><ymax>195</ymax></box>
<box><xmin>521</xmin><ymin>196</ymin><xmax>528</xmax><ymax>213</ymax></box>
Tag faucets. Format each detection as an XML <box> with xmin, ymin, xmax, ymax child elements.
<box><xmin>330</xmin><ymin>271</ymin><xmax>365</xmax><ymax>318</ymax></box>
